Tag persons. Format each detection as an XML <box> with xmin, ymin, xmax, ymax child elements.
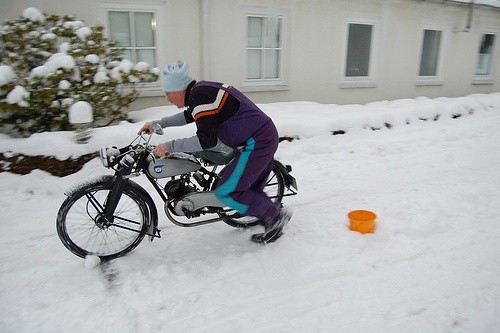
<box><xmin>137</xmin><ymin>58</ymin><xmax>292</xmax><ymax>243</ymax></box>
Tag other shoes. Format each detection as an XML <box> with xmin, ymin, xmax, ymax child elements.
<box><xmin>251</xmin><ymin>232</ymin><xmax>283</xmax><ymax>242</ymax></box>
<box><xmin>261</xmin><ymin>206</ymin><xmax>294</xmax><ymax>244</ymax></box>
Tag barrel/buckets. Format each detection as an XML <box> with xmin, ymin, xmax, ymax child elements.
<box><xmin>347</xmin><ymin>209</ymin><xmax>377</xmax><ymax>234</ymax></box>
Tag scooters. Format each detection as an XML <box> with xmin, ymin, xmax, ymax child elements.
<box><xmin>56</xmin><ymin>128</ymin><xmax>302</xmax><ymax>264</ymax></box>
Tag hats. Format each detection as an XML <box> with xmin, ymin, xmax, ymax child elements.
<box><xmin>162</xmin><ymin>60</ymin><xmax>193</xmax><ymax>92</ymax></box>
